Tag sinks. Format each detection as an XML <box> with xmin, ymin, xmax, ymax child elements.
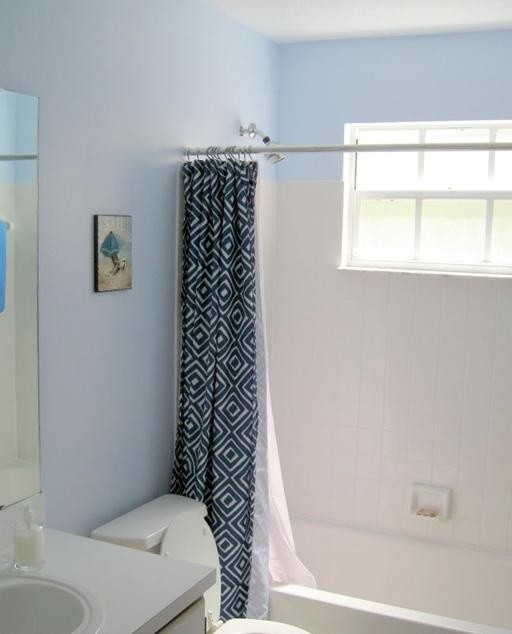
<box><xmin>0</xmin><ymin>567</ymin><xmax>103</xmax><ymax>634</ymax></box>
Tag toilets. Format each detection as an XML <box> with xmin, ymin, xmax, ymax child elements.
<box><xmin>91</xmin><ymin>494</ymin><xmax>308</xmax><ymax>634</ymax></box>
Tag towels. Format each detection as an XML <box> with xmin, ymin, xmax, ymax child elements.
<box><xmin>0</xmin><ymin>220</ymin><xmax>6</xmax><ymax>313</ymax></box>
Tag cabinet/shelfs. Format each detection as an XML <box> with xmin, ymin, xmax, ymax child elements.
<box><xmin>156</xmin><ymin>597</ymin><xmax>216</xmax><ymax>634</ymax></box>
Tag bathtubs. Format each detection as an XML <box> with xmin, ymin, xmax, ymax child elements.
<box><xmin>269</xmin><ymin>513</ymin><xmax>511</xmax><ymax>633</ymax></box>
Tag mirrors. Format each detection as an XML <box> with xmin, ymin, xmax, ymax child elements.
<box><xmin>0</xmin><ymin>87</ymin><xmax>43</xmax><ymax>511</ymax></box>
